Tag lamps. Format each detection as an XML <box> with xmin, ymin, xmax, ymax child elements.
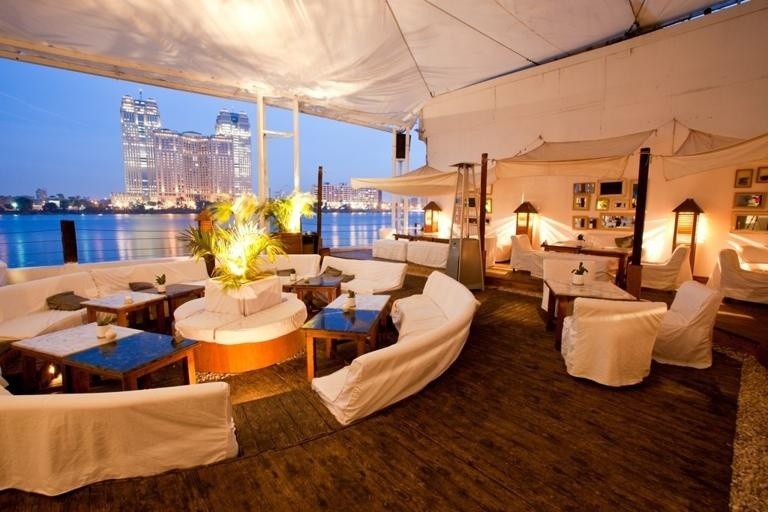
<box><xmin>670</xmin><ymin>196</ymin><xmax>706</xmax><ymax>279</ymax></box>
<box><xmin>513</xmin><ymin>200</ymin><xmax>539</xmax><ymax>248</ymax></box>
<box><xmin>422</xmin><ymin>200</ymin><xmax>443</xmax><ymax>235</ymax></box>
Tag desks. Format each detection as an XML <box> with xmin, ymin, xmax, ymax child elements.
<box><xmin>62</xmin><ymin>331</ymin><xmax>202</xmax><ymax>390</ymax></box>
<box><xmin>11</xmin><ymin>321</ymin><xmax>144</xmax><ymax>392</ymax></box>
<box><xmin>543</xmin><ymin>279</ymin><xmax>635</xmax><ymax>350</ymax></box>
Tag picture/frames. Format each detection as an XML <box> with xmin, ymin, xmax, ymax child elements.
<box><xmin>571</xmin><ymin>176</ymin><xmax>641</xmax><ymax>231</ymax></box>
<box><xmin>452</xmin><ymin>183</ymin><xmax>492</xmax><ymax>225</ymax></box>
<box><xmin>728</xmin><ymin>165</ymin><xmax>767</xmax><ymax>236</ymax></box>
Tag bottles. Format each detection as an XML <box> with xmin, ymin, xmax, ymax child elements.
<box><xmin>105</xmin><ymin>326</ymin><xmax>117</xmax><ymax>340</ymax></box>
<box><xmin>342</xmin><ymin>302</ymin><xmax>349</xmax><ymax>312</ymax></box>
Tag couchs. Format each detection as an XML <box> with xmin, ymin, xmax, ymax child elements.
<box><xmin>561</xmin><ymin>297</ymin><xmax>667</xmax><ymax>386</ymax></box>
<box><xmin>368</xmin><ymin>231</ymin><xmax>635</xmax><ymax>285</ymax></box>
<box><xmin>639</xmin><ymin>233</ymin><xmax>767</xmax><ymax>303</ymax></box>
<box><xmin>541</xmin><ymin>259</ymin><xmax>596</xmax><ymax>318</ymax></box>
<box><xmin>0</xmin><ymin>382</ymin><xmax>239</xmax><ymax>495</ymax></box>
<box><xmin>311</xmin><ymin>271</ymin><xmax>481</xmax><ymax>425</ymax></box>
<box><xmin>653</xmin><ymin>281</ymin><xmax>724</xmax><ymax>369</ymax></box>
<box><xmin>2</xmin><ymin>254</ymin><xmax>408</xmax><ymax>381</ymax></box>
